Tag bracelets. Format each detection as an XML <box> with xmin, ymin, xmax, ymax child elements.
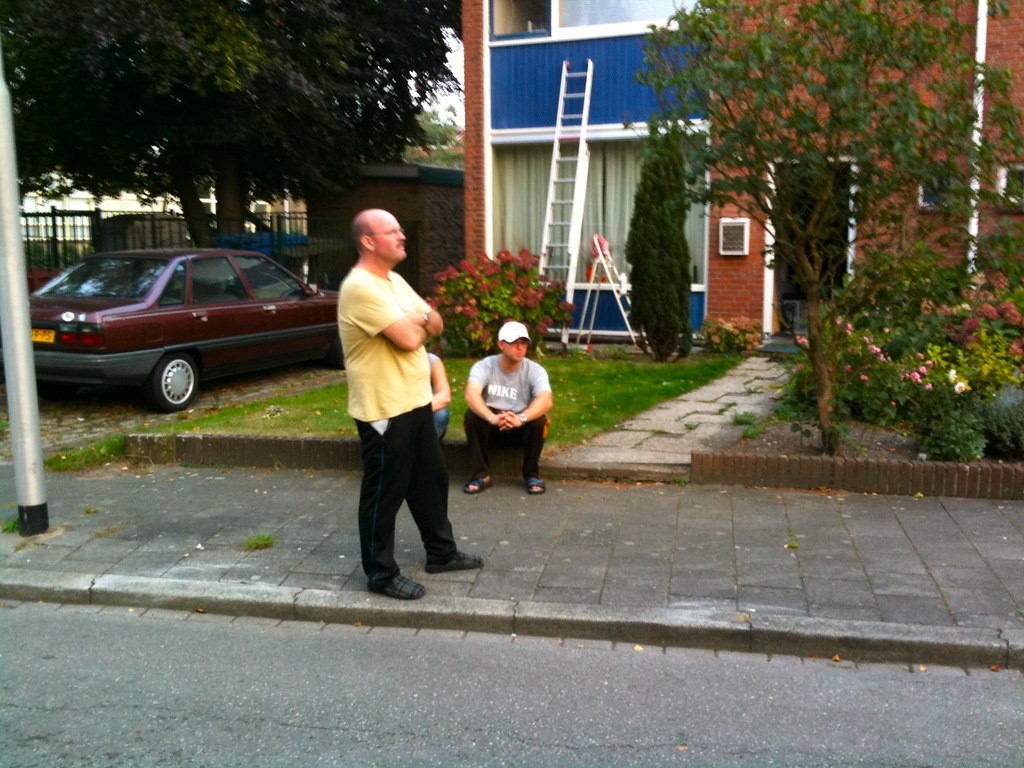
<box><xmin>488</xmin><ymin>413</ymin><xmax>495</xmax><ymax>423</ymax></box>
<box><xmin>422</xmin><ymin>313</ymin><xmax>428</xmax><ymax>321</ymax></box>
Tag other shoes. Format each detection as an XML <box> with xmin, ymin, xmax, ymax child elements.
<box><xmin>425</xmin><ymin>552</ymin><xmax>484</xmax><ymax>574</ymax></box>
<box><xmin>368</xmin><ymin>575</ymin><xmax>425</xmax><ymax>600</ymax></box>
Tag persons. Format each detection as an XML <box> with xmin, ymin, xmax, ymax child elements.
<box><xmin>463</xmin><ymin>321</ymin><xmax>553</xmax><ymax>494</ymax></box>
<box><xmin>338</xmin><ymin>209</ymin><xmax>485</xmax><ymax>599</ymax></box>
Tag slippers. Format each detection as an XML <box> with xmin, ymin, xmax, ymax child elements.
<box><xmin>464</xmin><ymin>478</ymin><xmax>493</xmax><ymax>494</ymax></box>
<box><xmin>527</xmin><ymin>478</ymin><xmax>546</xmax><ymax>494</ymax></box>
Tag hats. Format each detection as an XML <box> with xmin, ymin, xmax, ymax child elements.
<box><xmin>498</xmin><ymin>321</ymin><xmax>531</xmax><ymax>343</ymax></box>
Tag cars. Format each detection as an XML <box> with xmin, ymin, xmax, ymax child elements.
<box><xmin>0</xmin><ymin>247</ymin><xmax>345</xmax><ymax>414</ymax></box>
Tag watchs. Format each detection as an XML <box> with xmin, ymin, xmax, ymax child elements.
<box><xmin>519</xmin><ymin>414</ymin><xmax>525</xmax><ymax>424</ymax></box>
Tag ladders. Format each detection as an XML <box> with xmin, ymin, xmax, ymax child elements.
<box><xmin>575</xmin><ymin>236</ymin><xmax>650</xmax><ymax>355</ymax></box>
<box><xmin>532</xmin><ymin>58</ymin><xmax>593</xmax><ymax>348</ymax></box>
